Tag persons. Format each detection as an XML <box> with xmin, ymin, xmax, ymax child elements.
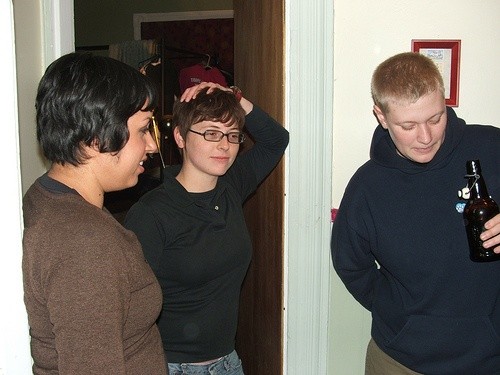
<box><xmin>119</xmin><ymin>81</ymin><xmax>289</xmax><ymax>375</ymax></box>
<box><xmin>331</xmin><ymin>52</ymin><xmax>499</xmax><ymax>375</ymax></box>
<box><xmin>21</xmin><ymin>51</ymin><xmax>170</xmax><ymax>375</ymax></box>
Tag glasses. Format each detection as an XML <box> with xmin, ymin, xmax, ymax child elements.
<box><xmin>187</xmin><ymin>128</ymin><xmax>247</xmax><ymax>143</ymax></box>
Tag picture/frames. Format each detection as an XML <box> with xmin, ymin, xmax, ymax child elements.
<box><xmin>412</xmin><ymin>38</ymin><xmax>461</xmax><ymax>107</ymax></box>
<box><xmin>132</xmin><ymin>10</ymin><xmax>235</xmax><ymax>88</ymax></box>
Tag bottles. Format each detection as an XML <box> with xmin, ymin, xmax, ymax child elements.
<box><xmin>463</xmin><ymin>160</ymin><xmax>500</xmax><ymax>262</ymax></box>
